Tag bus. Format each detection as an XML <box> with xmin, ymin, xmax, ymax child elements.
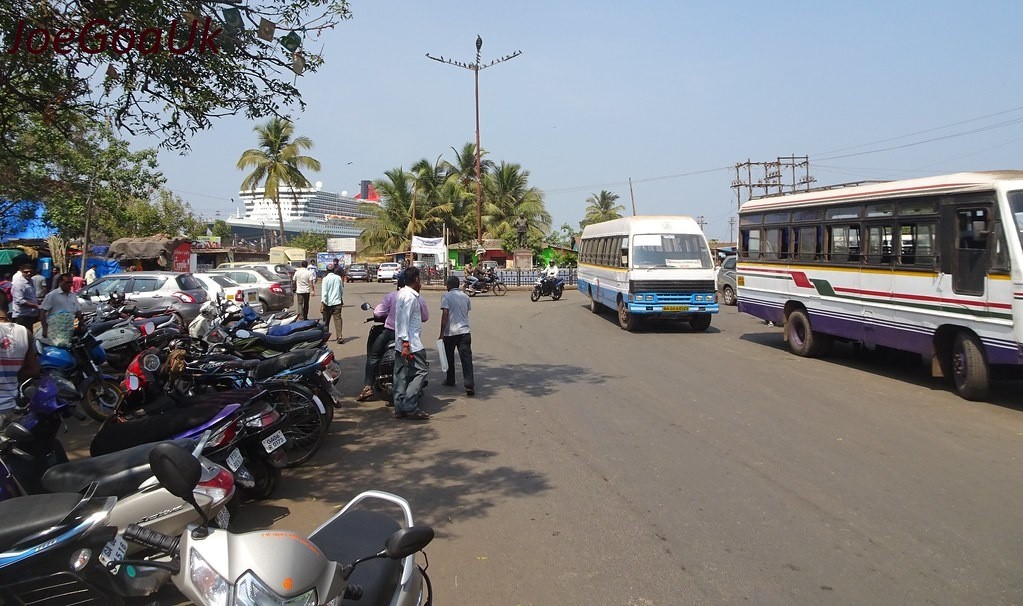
<box><xmin>736</xmin><ymin>171</ymin><xmax>1023</xmax><ymax>403</ymax></box>
<box><xmin>577</xmin><ymin>215</ymin><xmax>721</xmax><ymax>331</ymax></box>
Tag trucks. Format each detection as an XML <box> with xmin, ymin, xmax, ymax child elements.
<box><xmin>269</xmin><ymin>246</ymin><xmax>309</xmax><ymax>270</ymax></box>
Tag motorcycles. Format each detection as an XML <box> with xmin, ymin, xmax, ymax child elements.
<box><xmin>112</xmin><ymin>297</ymin><xmax>344</xmax><ymax>435</ymax></box>
<box><xmin>531</xmin><ymin>272</ymin><xmax>565</xmax><ymax>303</ymax></box>
<box><xmin>16</xmin><ymin>319</ymin><xmax>123</xmax><ymax>422</ymax></box>
<box><xmin>462</xmin><ymin>268</ymin><xmax>507</xmax><ymax>297</ymax></box>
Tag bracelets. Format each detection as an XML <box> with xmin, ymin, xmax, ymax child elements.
<box><xmin>403</xmin><ymin>343</ymin><xmax>409</xmax><ymax>346</ymax></box>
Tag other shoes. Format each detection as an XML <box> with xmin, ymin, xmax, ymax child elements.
<box><xmin>406</xmin><ymin>407</ymin><xmax>429</xmax><ymax>418</ymax></box>
<box><xmin>443</xmin><ymin>379</ymin><xmax>456</xmax><ymax>386</ymax></box>
<box><xmin>466</xmin><ymin>388</ymin><xmax>477</xmax><ymax>396</ymax></box>
<box><xmin>396</xmin><ymin>411</ymin><xmax>406</xmax><ymax>418</ymax></box>
<box><xmin>337</xmin><ymin>337</ymin><xmax>344</xmax><ymax>344</ymax></box>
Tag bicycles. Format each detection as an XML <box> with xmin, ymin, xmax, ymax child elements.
<box><xmin>162</xmin><ymin>339</ymin><xmax>326</xmax><ymax>470</ymax></box>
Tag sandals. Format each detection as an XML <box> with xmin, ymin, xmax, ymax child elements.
<box><xmin>357</xmin><ymin>386</ymin><xmax>374</xmax><ymax>401</ymax></box>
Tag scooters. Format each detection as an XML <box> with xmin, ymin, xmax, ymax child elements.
<box><xmin>0</xmin><ymin>345</ymin><xmax>283</xmax><ymax>606</ymax></box>
<box><xmin>359</xmin><ymin>302</ymin><xmax>430</xmax><ymax>400</ymax></box>
<box><xmin>33</xmin><ymin>287</ymin><xmax>185</xmax><ymax>373</ymax></box>
<box><xmin>104</xmin><ymin>444</ymin><xmax>437</xmax><ymax>606</ymax></box>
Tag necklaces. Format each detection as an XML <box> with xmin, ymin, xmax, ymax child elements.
<box><xmin>0</xmin><ymin>317</ymin><xmax>9</xmax><ymax>322</ymax></box>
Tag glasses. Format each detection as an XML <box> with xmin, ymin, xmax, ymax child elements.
<box><xmin>22</xmin><ymin>270</ymin><xmax>34</xmax><ymax>275</ymax></box>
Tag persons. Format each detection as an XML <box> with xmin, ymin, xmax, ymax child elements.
<box><xmin>70</xmin><ymin>270</ymin><xmax>83</xmax><ymax>292</ymax></box>
<box><xmin>40</xmin><ymin>274</ymin><xmax>86</xmax><ymax>338</ymax></box>
<box><xmin>0</xmin><ymin>264</ymin><xmax>47</xmax><ymax>329</ymax></box>
<box><xmin>293</xmin><ymin>261</ymin><xmax>315</xmax><ymax>320</ymax></box>
<box><xmin>356</xmin><ymin>275</ymin><xmax>429</xmax><ymax>402</ymax></box>
<box><xmin>466</xmin><ymin>260</ymin><xmax>491</xmax><ymax>292</ymax></box>
<box><xmin>515</xmin><ymin>213</ymin><xmax>529</xmax><ymax>249</ymax></box>
<box><xmin>0</xmin><ymin>290</ymin><xmax>40</xmax><ymax>431</ymax></box>
<box><xmin>85</xmin><ymin>264</ymin><xmax>97</xmax><ymax>285</ymax></box>
<box><xmin>320</xmin><ymin>264</ymin><xmax>343</xmax><ymax>344</ymax></box>
<box><xmin>438</xmin><ymin>275</ymin><xmax>474</xmax><ymax>394</ymax></box>
<box><xmin>542</xmin><ymin>260</ymin><xmax>559</xmax><ymax>297</ymax></box>
<box><xmin>394</xmin><ymin>267</ymin><xmax>429</xmax><ymax>418</ymax></box>
<box><xmin>307</xmin><ymin>260</ymin><xmax>318</xmax><ymax>296</ymax></box>
<box><xmin>50</xmin><ymin>267</ymin><xmax>62</xmax><ymax>290</ymax></box>
<box><xmin>333</xmin><ymin>259</ymin><xmax>346</xmax><ymax>285</ymax></box>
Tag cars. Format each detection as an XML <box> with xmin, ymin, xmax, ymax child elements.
<box><xmin>73</xmin><ymin>271</ymin><xmax>211</xmax><ymax>335</ymax></box>
<box><xmin>207</xmin><ymin>261</ymin><xmax>298</xmax><ymax>318</ymax></box>
<box><xmin>716</xmin><ymin>254</ymin><xmax>738</xmax><ymax>305</ymax></box>
<box><xmin>376</xmin><ymin>263</ymin><xmax>400</xmax><ymax>284</ymax></box>
<box><xmin>346</xmin><ymin>263</ymin><xmax>373</xmax><ymax>283</ymax></box>
<box><xmin>368</xmin><ymin>264</ymin><xmax>380</xmax><ymax>278</ymax></box>
<box><xmin>192</xmin><ymin>273</ymin><xmax>263</xmax><ymax>316</ymax></box>
<box><xmin>412</xmin><ymin>260</ymin><xmax>430</xmax><ymax>273</ymax></box>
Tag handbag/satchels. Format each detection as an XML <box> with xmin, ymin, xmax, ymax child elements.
<box><xmin>436</xmin><ymin>339</ymin><xmax>449</xmax><ymax>373</ymax></box>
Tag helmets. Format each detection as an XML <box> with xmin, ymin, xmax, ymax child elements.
<box><xmin>549</xmin><ymin>259</ymin><xmax>555</xmax><ymax>266</ymax></box>
